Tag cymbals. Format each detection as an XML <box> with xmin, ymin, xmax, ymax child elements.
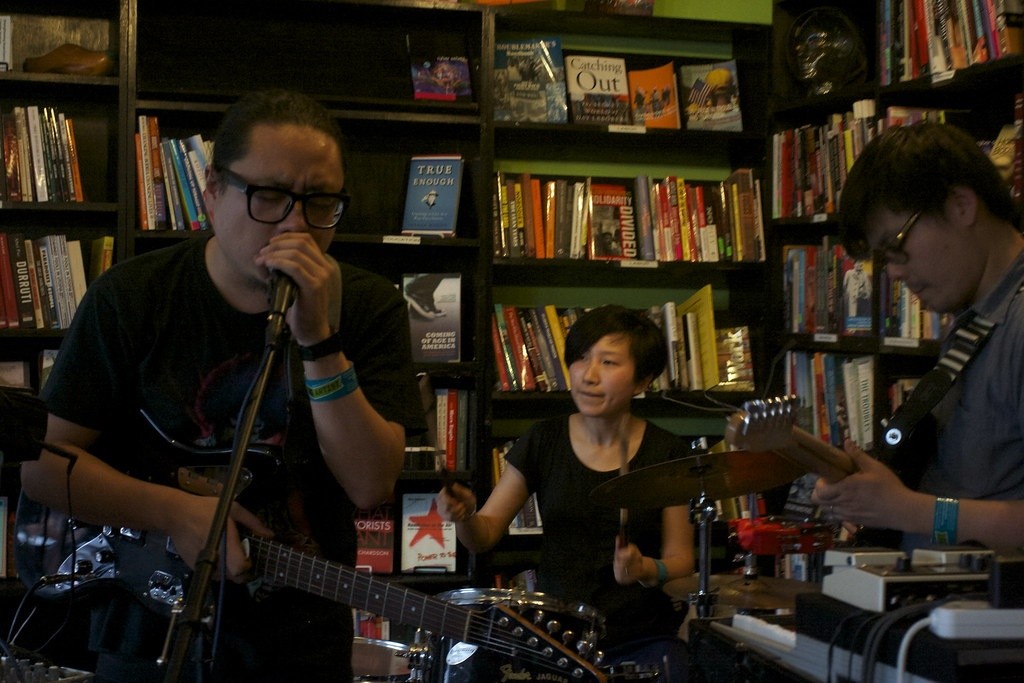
<box><xmin>589</xmin><ymin>447</ymin><xmax>809</xmax><ymax>510</ymax></box>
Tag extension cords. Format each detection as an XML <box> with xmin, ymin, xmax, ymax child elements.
<box><xmin>928</xmin><ymin>601</ymin><xmax>1024</xmax><ymax>641</ymax></box>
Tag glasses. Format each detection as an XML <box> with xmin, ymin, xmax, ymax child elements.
<box><xmin>883</xmin><ymin>211</ymin><xmax>922</xmax><ymax>266</ymax></box>
<box><xmin>221</xmin><ymin>166</ymin><xmax>351</xmax><ymax>229</ymax></box>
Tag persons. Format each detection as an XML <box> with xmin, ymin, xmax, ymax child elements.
<box><xmin>437</xmin><ymin>304</ymin><xmax>694</xmax><ymax>682</ymax></box>
<box><xmin>809</xmin><ymin>118</ymin><xmax>1024</xmax><ymax>558</ymax></box>
<box><xmin>22</xmin><ymin>87</ymin><xmax>429</xmax><ymax>682</ymax></box>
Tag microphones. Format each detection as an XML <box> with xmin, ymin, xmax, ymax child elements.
<box><xmin>264</xmin><ymin>270</ymin><xmax>296</xmax><ymax>351</ymax></box>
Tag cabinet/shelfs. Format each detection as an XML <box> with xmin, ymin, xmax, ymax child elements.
<box><xmin>773</xmin><ymin>0</ymin><xmax>1024</xmax><ymax>515</ymax></box>
<box><xmin>0</xmin><ymin>0</ymin><xmax>772</xmax><ymax>594</ymax></box>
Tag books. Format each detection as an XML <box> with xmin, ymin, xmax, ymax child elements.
<box><xmin>0</xmin><ymin>2</ymin><xmax>1024</xmax><ymax>585</ymax></box>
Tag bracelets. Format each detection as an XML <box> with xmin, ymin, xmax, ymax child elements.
<box><xmin>305</xmin><ymin>362</ymin><xmax>360</xmax><ymax>402</ymax></box>
<box><xmin>652</xmin><ymin>556</ymin><xmax>668</xmax><ymax>584</ymax></box>
<box><xmin>929</xmin><ymin>494</ymin><xmax>959</xmax><ymax>547</ymax></box>
<box><xmin>298</xmin><ymin>332</ymin><xmax>342</xmax><ymax>362</ymax></box>
<box><xmin>463</xmin><ymin>505</ymin><xmax>477</xmax><ymax>522</ymax></box>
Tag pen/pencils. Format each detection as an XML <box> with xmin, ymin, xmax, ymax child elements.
<box><xmin>737</xmin><ymin>608</ymin><xmax>791</xmax><ymax>615</ymax></box>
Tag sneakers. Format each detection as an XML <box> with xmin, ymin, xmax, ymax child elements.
<box><xmin>405</xmin><ymin>292</ymin><xmax>447</xmax><ymax>319</ymax></box>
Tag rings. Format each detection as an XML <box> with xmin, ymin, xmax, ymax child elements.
<box><xmin>830</xmin><ymin>504</ymin><xmax>835</xmax><ymax>518</ymax></box>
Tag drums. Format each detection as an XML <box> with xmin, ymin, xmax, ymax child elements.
<box><xmin>351</xmin><ymin>635</ymin><xmax>415</xmax><ymax>683</ymax></box>
<box><xmin>418</xmin><ymin>585</ymin><xmax>609</xmax><ymax>683</ymax></box>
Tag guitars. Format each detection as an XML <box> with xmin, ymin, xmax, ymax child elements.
<box><xmin>9</xmin><ymin>400</ymin><xmax>608</xmax><ymax>683</ymax></box>
<box><xmin>723</xmin><ymin>393</ymin><xmax>940</xmax><ymax>547</ymax></box>
